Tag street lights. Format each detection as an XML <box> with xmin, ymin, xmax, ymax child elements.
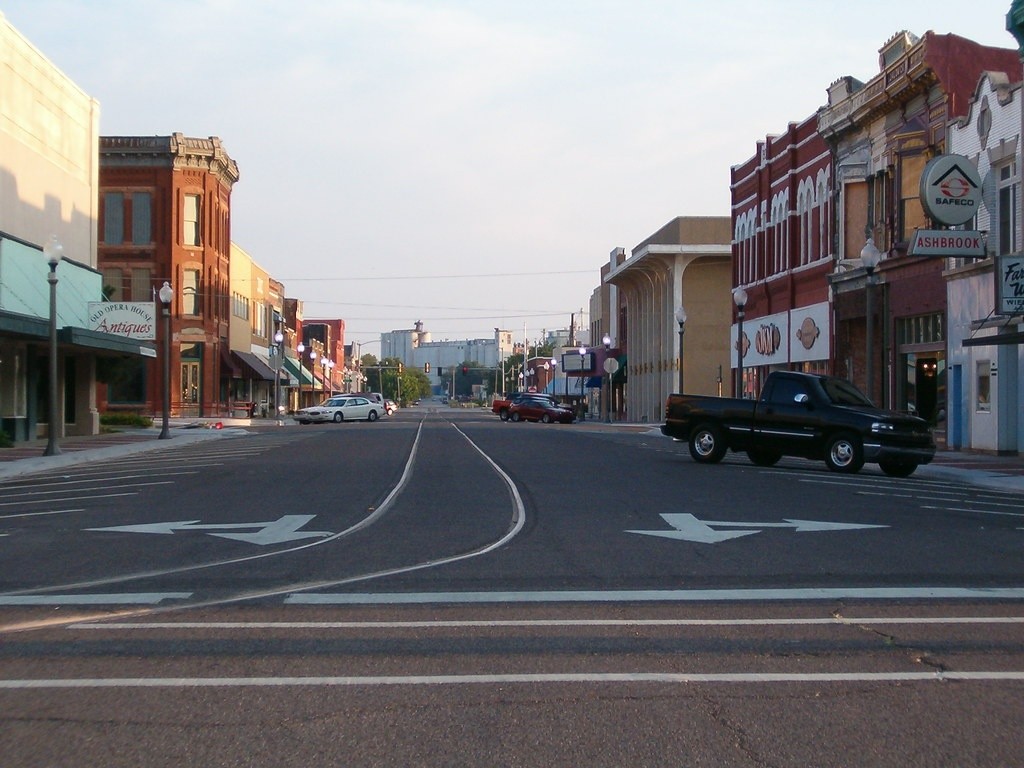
<box><xmin>363</xmin><ymin>376</ymin><xmax>368</xmax><ymax>393</ymax></box>
<box><xmin>328</xmin><ymin>360</ymin><xmax>334</xmax><ymax>397</ymax></box>
<box><xmin>525</xmin><ymin>369</ymin><xmax>530</xmax><ymax>392</ymax></box>
<box><xmin>506</xmin><ymin>377</ymin><xmax>509</xmax><ymax>392</ymax></box>
<box><xmin>159</xmin><ymin>281</ymin><xmax>175</xmax><ymax>439</ymax></box>
<box><xmin>43</xmin><ymin>233</ymin><xmax>65</xmax><ymax>456</ymax></box>
<box><xmin>511</xmin><ymin>376</ymin><xmax>515</xmax><ymax>393</ymax></box>
<box><xmin>530</xmin><ymin>368</ymin><xmax>535</xmax><ymax>389</ymax></box>
<box><xmin>357</xmin><ymin>339</ymin><xmax>391</xmax><ymax>392</ymax></box>
<box><xmin>675</xmin><ymin>307</ymin><xmax>688</xmax><ymax>394</ymax></box>
<box><xmin>446</xmin><ymin>381</ymin><xmax>450</xmax><ymax>402</ymax></box>
<box><xmin>398</xmin><ymin>363</ymin><xmax>403</xmax><ymax>373</ymax></box>
<box><xmin>551</xmin><ymin>359</ymin><xmax>557</xmax><ymax>395</ymax></box>
<box><xmin>275</xmin><ymin>330</ymin><xmax>284</xmax><ymax>420</ymax></box>
<box><xmin>356</xmin><ymin>373</ymin><xmax>359</xmax><ymax>393</ymax></box>
<box><xmin>309</xmin><ymin>350</ymin><xmax>317</xmax><ymax>407</ymax></box>
<box><xmin>544</xmin><ymin>362</ymin><xmax>550</xmax><ymax>394</ymax></box>
<box><xmin>506</xmin><ymin>392</ymin><xmax>523</xmax><ymax>401</ymax></box>
<box><xmin>348</xmin><ymin>368</ymin><xmax>352</xmax><ymax>393</ymax></box>
<box><xmin>734</xmin><ymin>287</ymin><xmax>748</xmax><ymax>399</ymax></box>
<box><xmin>397</xmin><ymin>376</ymin><xmax>402</xmax><ymax>406</ymax></box>
<box><xmin>425</xmin><ymin>363</ymin><xmax>430</xmax><ymax>373</ymax></box>
<box><xmin>861</xmin><ymin>238</ymin><xmax>880</xmax><ymax>402</ymax></box>
<box><xmin>463</xmin><ymin>366</ymin><xmax>467</xmax><ymax>376</ymax></box>
<box><xmin>518</xmin><ymin>372</ymin><xmax>524</xmax><ymax>393</ymax></box>
<box><xmin>603</xmin><ymin>332</ymin><xmax>611</xmax><ymax>422</ymax></box>
<box><xmin>321</xmin><ymin>356</ymin><xmax>328</xmax><ymax>401</ymax></box>
<box><xmin>297</xmin><ymin>342</ymin><xmax>305</xmax><ymax>409</ymax></box>
<box><xmin>578</xmin><ymin>344</ymin><xmax>587</xmax><ymax>422</ymax></box>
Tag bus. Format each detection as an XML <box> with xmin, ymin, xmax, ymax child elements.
<box><xmin>440</xmin><ymin>396</ymin><xmax>448</xmax><ymax>402</ymax></box>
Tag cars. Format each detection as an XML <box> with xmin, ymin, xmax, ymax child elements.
<box><xmin>510</xmin><ymin>397</ymin><xmax>573</xmax><ymax>414</ymax></box>
<box><xmin>333</xmin><ymin>393</ymin><xmax>379</xmax><ymax>404</ymax></box>
<box><xmin>418</xmin><ymin>398</ymin><xmax>422</xmax><ymax>401</ymax></box>
<box><xmin>292</xmin><ymin>398</ymin><xmax>337</xmax><ymax>425</ymax></box>
<box><xmin>443</xmin><ymin>401</ymin><xmax>448</xmax><ymax>404</ymax></box>
<box><xmin>383</xmin><ymin>399</ymin><xmax>398</xmax><ymax>416</ymax></box>
<box><xmin>413</xmin><ymin>400</ymin><xmax>419</xmax><ymax>406</ymax></box>
<box><xmin>308</xmin><ymin>396</ymin><xmax>384</xmax><ymax>424</ymax></box>
<box><xmin>509</xmin><ymin>397</ymin><xmax>573</xmax><ymax>424</ymax></box>
<box><xmin>432</xmin><ymin>398</ymin><xmax>436</xmax><ymax>401</ymax></box>
<box><xmin>393</xmin><ymin>401</ymin><xmax>399</xmax><ymax>414</ymax></box>
<box><xmin>372</xmin><ymin>393</ymin><xmax>384</xmax><ymax>407</ymax></box>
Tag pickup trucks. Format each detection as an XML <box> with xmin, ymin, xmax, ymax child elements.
<box><xmin>491</xmin><ymin>393</ymin><xmax>560</xmax><ymax>421</ymax></box>
<box><xmin>659</xmin><ymin>370</ymin><xmax>938</xmax><ymax>477</ymax></box>
